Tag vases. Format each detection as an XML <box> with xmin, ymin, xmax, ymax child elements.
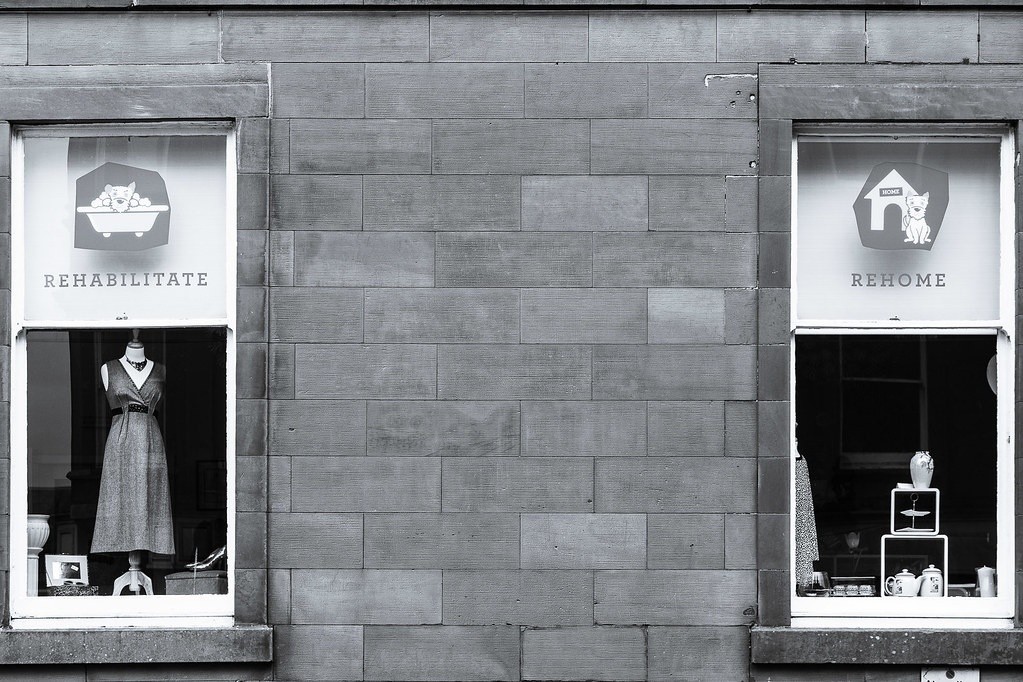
<box><xmin>909</xmin><ymin>451</ymin><xmax>935</xmax><ymax>488</ymax></box>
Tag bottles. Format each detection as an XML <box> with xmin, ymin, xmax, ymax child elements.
<box><xmin>920</xmin><ymin>564</ymin><xmax>944</xmax><ymax>597</ymax></box>
<box><xmin>910</xmin><ymin>451</ymin><xmax>934</xmax><ymax>488</ymax></box>
<box><xmin>798</xmin><ymin>572</ymin><xmax>831</xmax><ymax>597</ymax></box>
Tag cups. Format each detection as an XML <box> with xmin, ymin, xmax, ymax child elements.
<box><xmin>833</xmin><ymin>584</ymin><xmax>872</xmax><ymax>596</ymax></box>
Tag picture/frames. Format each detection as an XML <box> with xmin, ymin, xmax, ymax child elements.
<box><xmin>45</xmin><ymin>554</ymin><xmax>89</xmax><ymax>586</ymax></box>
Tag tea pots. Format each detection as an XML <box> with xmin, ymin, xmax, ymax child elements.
<box><xmin>886</xmin><ymin>569</ymin><xmax>926</xmax><ymax>597</ymax></box>
<box><xmin>844</xmin><ymin>532</ymin><xmax>861</xmax><ymax>548</ymax></box>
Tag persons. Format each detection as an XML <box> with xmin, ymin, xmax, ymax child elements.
<box><xmin>90</xmin><ymin>341</ymin><xmax>176</xmax><ymax>555</ymax></box>
